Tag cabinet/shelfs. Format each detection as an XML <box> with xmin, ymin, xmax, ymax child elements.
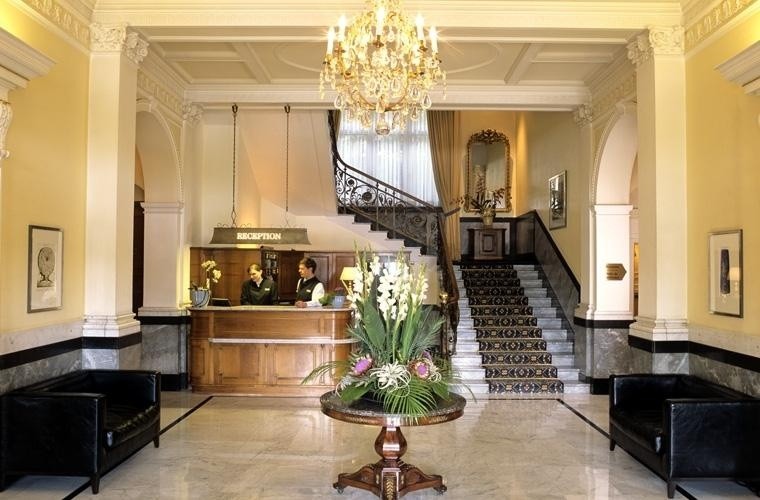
<box><xmin>189</xmin><ymin>247</ymin><xmax>413</xmax><ymax>307</ymax></box>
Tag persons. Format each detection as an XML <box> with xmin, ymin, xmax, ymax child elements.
<box><xmin>294</xmin><ymin>256</ymin><xmax>326</xmax><ymax>308</ymax></box>
<box><xmin>239</xmin><ymin>263</ymin><xmax>279</xmax><ymax>305</ymax></box>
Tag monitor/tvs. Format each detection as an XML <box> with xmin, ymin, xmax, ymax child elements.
<box><xmin>211</xmin><ymin>298</ymin><xmax>232</xmax><ymax>306</ymax></box>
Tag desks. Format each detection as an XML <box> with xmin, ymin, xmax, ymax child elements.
<box><xmin>319</xmin><ymin>386</ymin><xmax>469</xmax><ymax>499</ymax></box>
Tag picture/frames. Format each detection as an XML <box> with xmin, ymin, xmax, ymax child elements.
<box><xmin>707</xmin><ymin>228</ymin><xmax>744</xmax><ymax>318</ymax></box>
<box><xmin>548</xmin><ymin>169</ymin><xmax>567</xmax><ymax>231</ymax></box>
<box><xmin>27</xmin><ymin>224</ymin><xmax>64</xmax><ymax>314</ymax></box>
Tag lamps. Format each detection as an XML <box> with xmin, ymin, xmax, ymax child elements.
<box><xmin>314</xmin><ymin>0</ymin><xmax>453</xmax><ymax>137</ymax></box>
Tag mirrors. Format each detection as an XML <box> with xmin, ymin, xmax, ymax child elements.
<box><xmin>464</xmin><ymin>128</ymin><xmax>513</xmax><ymax>214</ymax></box>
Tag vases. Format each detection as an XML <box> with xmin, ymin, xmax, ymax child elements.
<box><xmin>480</xmin><ymin>208</ymin><xmax>497</xmax><ymax>225</ymax></box>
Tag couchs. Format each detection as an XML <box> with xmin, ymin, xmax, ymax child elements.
<box><xmin>1</xmin><ymin>369</ymin><xmax>161</xmax><ymax>495</ymax></box>
<box><xmin>608</xmin><ymin>373</ymin><xmax>759</xmax><ymax>499</ymax></box>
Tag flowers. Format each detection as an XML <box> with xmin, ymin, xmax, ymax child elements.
<box><xmin>450</xmin><ymin>175</ymin><xmax>511</xmax><ymax>215</ymax></box>
<box><xmin>300</xmin><ymin>235</ymin><xmax>478</xmax><ymax>425</ymax></box>
